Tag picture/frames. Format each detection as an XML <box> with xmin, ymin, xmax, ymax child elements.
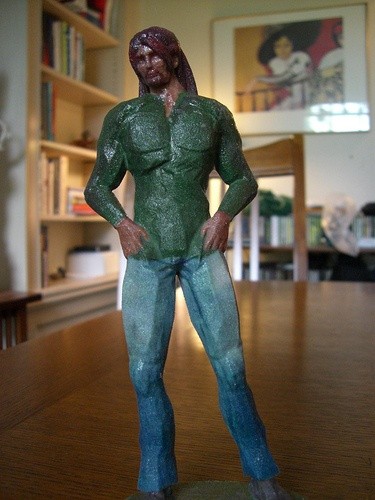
<box><xmin>210</xmin><ymin>0</ymin><xmax>375</xmax><ymax>139</ymax></box>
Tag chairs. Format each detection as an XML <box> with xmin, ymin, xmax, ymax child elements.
<box><xmin>208</xmin><ymin>133</ymin><xmax>307</xmax><ymax>284</ymax></box>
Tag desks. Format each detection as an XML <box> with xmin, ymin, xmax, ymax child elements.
<box><xmin>0</xmin><ymin>279</ymin><xmax>375</xmax><ymax>500</ymax></box>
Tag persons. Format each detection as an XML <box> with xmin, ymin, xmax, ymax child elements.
<box><xmin>84</xmin><ymin>27</ymin><xmax>292</xmax><ymax>500</ymax></box>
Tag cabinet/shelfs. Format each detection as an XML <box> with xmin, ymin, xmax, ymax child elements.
<box><xmin>27</xmin><ymin>0</ymin><xmax>146</xmax><ymax>339</ymax></box>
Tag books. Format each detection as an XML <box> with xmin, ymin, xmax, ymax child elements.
<box><xmin>40</xmin><ymin>151</ymin><xmax>99</xmax><ymax>216</ymax></box>
<box><xmin>261</xmin><ymin>215</ymin><xmax>375</xmax><ymax>247</ymax></box>
<box><xmin>42</xmin><ymin>81</ymin><xmax>58</xmax><ymax>141</ymax></box>
<box><xmin>44</xmin><ymin>0</ymin><xmax>123</xmax><ymax>80</ymax></box>
